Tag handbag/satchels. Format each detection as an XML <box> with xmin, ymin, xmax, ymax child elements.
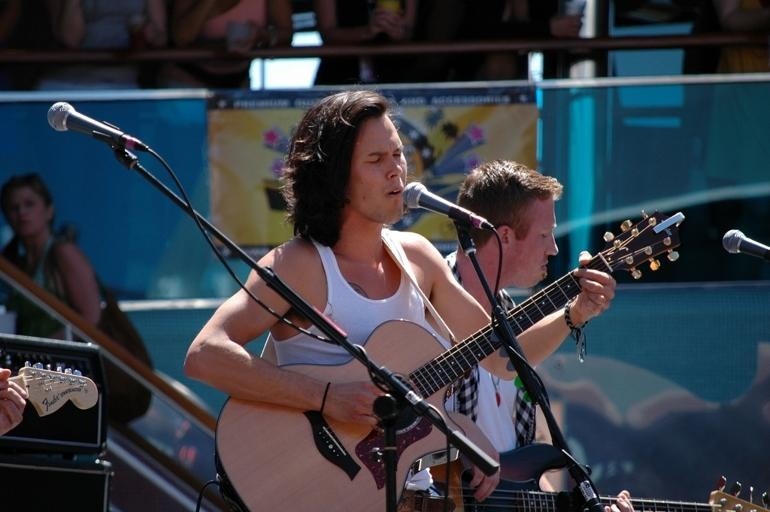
<box><xmin>96</xmin><ymin>307</ymin><xmax>155</xmax><ymax>422</ymax></box>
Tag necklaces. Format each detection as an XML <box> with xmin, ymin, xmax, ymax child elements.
<box><xmin>488</xmin><ymin>372</ymin><xmax>502</xmax><ymax>408</ymax></box>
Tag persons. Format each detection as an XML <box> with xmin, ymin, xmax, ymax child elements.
<box><xmin>0</xmin><ymin>369</ymin><xmax>29</xmax><ymax>440</ymax></box>
<box><xmin>416</xmin><ymin>158</ymin><xmax>637</xmax><ymax>512</ymax></box>
<box><xmin>183</xmin><ymin>93</ymin><xmax>619</xmax><ymax>511</ymax></box>
<box><xmin>1</xmin><ymin>174</ymin><xmax>157</xmax><ymax>423</ymax></box>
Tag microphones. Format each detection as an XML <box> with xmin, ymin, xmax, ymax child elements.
<box><xmin>46</xmin><ymin>101</ymin><xmax>150</xmax><ymax>153</ymax></box>
<box><xmin>401</xmin><ymin>180</ymin><xmax>496</xmax><ymax>233</ymax></box>
<box><xmin>722</xmin><ymin>229</ymin><xmax>770</xmax><ymax>260</ymax></box>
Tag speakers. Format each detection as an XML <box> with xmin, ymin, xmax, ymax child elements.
<box><xmin>0</xmin><ymin>456</ymin><xmax>114</xmax><ymax>512</ymax></box>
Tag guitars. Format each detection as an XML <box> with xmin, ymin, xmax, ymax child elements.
<box><xmin>8</xmin><ymin>361</ymin><xmax>98</xmax><ymax>416</ymax></box>
<box><xmin>396</xmin><ymin>443</ymin><xmax>770</xmax><ymax>512</ymax></box>
<box><xmin>214</xmin><ymin>209</ymin><xmax>685</xmax><ymax>510</ymax></box>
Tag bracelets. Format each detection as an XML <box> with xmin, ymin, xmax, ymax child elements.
<box><xmin>564</xmin><ymin>298</ymin><xmax>588</xmax><ymax>334</ymax></box>
<box><xmin>319</xmin><ymin>382</ymin><xmax>331</xmax><ymax>414</ymax></box>
